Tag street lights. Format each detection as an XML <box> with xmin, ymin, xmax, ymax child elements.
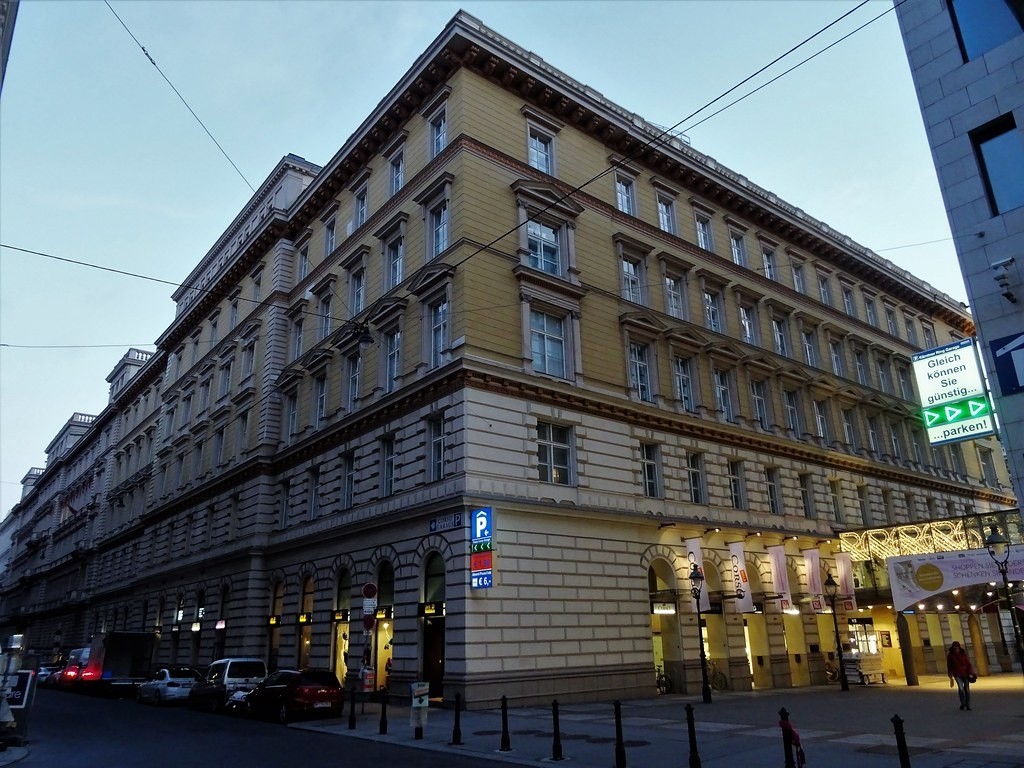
<box><xmin>824</xmin><ymin>575</ymin><xmax>849</xmax><ymax>691</ymax></box>
<box><xmin>690</xmin><ymin>564</ymin><xmax>712</xmax><ymax>702</ymax></box>
<box><xmin>983</xmin><ymin>525</ymin><xmax>1024</xmax><ymax>677</ymax></box>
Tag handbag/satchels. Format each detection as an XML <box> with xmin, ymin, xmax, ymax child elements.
<box><xmin>968</xmin><ymin>672</ymin><xmax>977</xmax><ymax>683</ymax></box>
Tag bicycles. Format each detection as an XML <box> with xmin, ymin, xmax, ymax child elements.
<box><xmin>656</xmin><ymin>665</ymin><xmax>672</xmax><ymax>694</ymax></box>
<box><xmin>825</xmin><ymin>661</ymin><xmax>840</xmax><ymax>683</ymax></box>
<box><xmin>707</xmin><ymin>660</ymin><xmax>727</xmax><ymax>691</ymax></box>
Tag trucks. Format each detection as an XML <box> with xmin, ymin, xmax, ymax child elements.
<box><xmin>80</xmin><ymin>631</ymin><xmax>156</xmax><ymax>697</ymax></box>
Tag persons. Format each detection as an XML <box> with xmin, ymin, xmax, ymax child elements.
<box><xmin>385</xmin><ymin>658</ymin><xmax>392</xmax><ymax>675</ymax></box>
<box><xmin>947</xmin><ymin>641</ymin><xmax>974</xmax><ymax>709</ymax></box>
<box><xmin>358</xmin><ymin>657</ymin><xmax>374</xmax><ymax>678</ymax></box>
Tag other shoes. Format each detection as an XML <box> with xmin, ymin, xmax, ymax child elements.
<box><xmin>959</xmin><ymin>704</ymin><xmax>965</xmax><ymax>709</ymax></box>
<box><xmin>967</xmin><ymin>706</ymin><xmax>972</xmax><ymax>711</ymax></box>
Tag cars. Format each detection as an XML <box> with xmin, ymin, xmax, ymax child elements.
<box><xmin>246</xmin><ymin>666</ymin><xmax>344</xmax><ymax>721</ymax></box>
<box><xmin>136</xmin><ymin>667</ymin><xmax>202</xmax><ymax>707</ymax></box>
<box><xmin>38</xmin><ymin>665</ymin><xmax>65</xmax><ymax>690</ymax></box>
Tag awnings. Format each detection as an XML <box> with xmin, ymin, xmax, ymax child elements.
<box><xmin>1016</xmin><ymin>605</ymin><xmax>1024</xmax><ymax>613</ymax></box>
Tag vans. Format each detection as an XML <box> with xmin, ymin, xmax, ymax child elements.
<box><xmin>188</xmin><ymin>658</ymin><xmax>268</xmax><ymax>714</ymax></box>
<box><xmin>61</xmin><ymin>648</ymin><xmax>91</xmax><ymax>692</ymax></box>
